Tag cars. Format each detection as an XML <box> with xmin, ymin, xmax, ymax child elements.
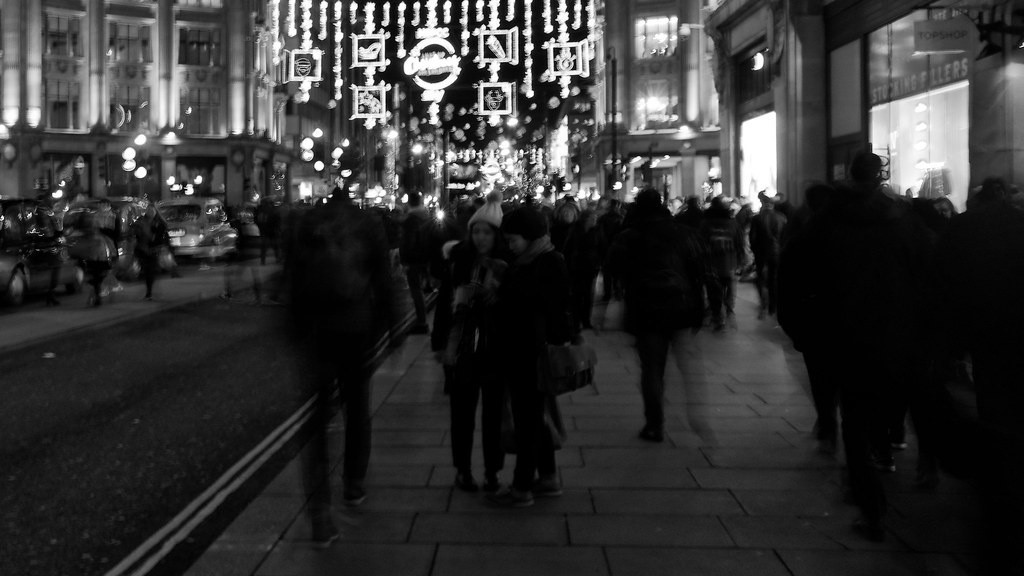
<box><xmin>62</xmin><ymin>197</ymin><xmax>175</xmax><ymax>278</ymax></box>
<box><xmin>156</xmin><ymin>197</ymin><xmax>237</xmax><ymax>262</ymax></box>
<box><xmin>0</xmin><ymin>196</ymin><xmax>87</xmax><ymax>303</ymax></box>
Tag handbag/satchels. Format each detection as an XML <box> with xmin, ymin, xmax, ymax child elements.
<box><xmin>540</xmin><ymin>334</ymin><xmax>597</xmax><ymax>396</ymax></box>
<box><xmin>434</xmin><ymin>262</ymin><xmax>497</xmax><ymax>366</ymax></box>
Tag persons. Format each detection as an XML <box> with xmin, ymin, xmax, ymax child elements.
<box><xmin>283</xmin><ymin>189</ymin><xmax>405</xmax><ymax>548</ymax></box>
<box><xmin>431</xmin><ymin>201</ymin><xmax>509</xmax><ymax>493</ymax></box>
<box><xmin>128</xmin><ymin>194</ymin><xmax>170</xmax><ymax>298</ymax></box>
<box><xmin>217</xmin><ymin>187</ymin><xmax>795</xmax><ymax>335</ymax></box>
<box><xmin>602</xmin><ymin>188</ymin><xmax>711</xmax><ymax>442</ymax></box>
<box><xmin>485</xmin><ymin>208</ymin><xmax>582</xmax><ymax>508</ymax></box>
<box><xmin>82</xmin><ymin>199</ymin><xmax>120</xmax><ymax>305</ymax></box>
<box><xmin>775</xmin><ymin>154</ymin><xmax>1024</xmax><ymax>576</ymax></box>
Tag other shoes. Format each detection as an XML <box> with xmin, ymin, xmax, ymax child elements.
<box><xmin>482</xmin><ymin>487</ymin><xmax>534</xmax><ymax>507</ymax></box>
<box><xmin>484</xmin><ymin>477</ymin><xmax>501</xmax><ymax>491</ymax></box>
<box><xmin>311</xmin><ymin>528</ymin><xmax>339</xmax><ymax>548</ymax></box>
<box><xmin>455</xmin><ymin>472</ymin><xmax>480</xmax><ymax>494</ymax></box>
<box><xmin>912</xmin><ymin>472</ymin><xmax>935</xmax><ymax>487</ymax></box>
<box><xmin>639</xmin><ymin>425</ymin><xmax>664</xmax><ymax>441</ymax></box>
<box><xmin>820</xmin><ymin>440</ymin><xmax>835</xmax><ymax>453</ymax></box>
<box><xmin>854</xmin><ymin>518</ymin><xmax>886</xmax><ymax>542</ymax></box>
<box><xmin>871</xmin><ymin>456</ymin><xmax>896</xmax><ymax>472</ymax></box>
<box><xmin>345</xmin><ymin>488</ymin><xmax>366</xmax><ymax>506</ymax></box>
<box><xmin>531</xmin><ymin>477</ymin><xmax>563</xmax><ymax>496</ymax></box>
<box><xmin>889</xmin><ymin>433</ymin><xmax>907</xmax><ymax>448</ymax></box>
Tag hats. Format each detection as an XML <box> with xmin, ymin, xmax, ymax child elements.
<box><xmin>467</xmin><ymin>191</ymin><xmax>504</xmax><ymax>234</ymax></box>
<box><xmin>500</xmin><ymin>207</ymin><xmax>547</xmax><ymax>235</ymax></box>
